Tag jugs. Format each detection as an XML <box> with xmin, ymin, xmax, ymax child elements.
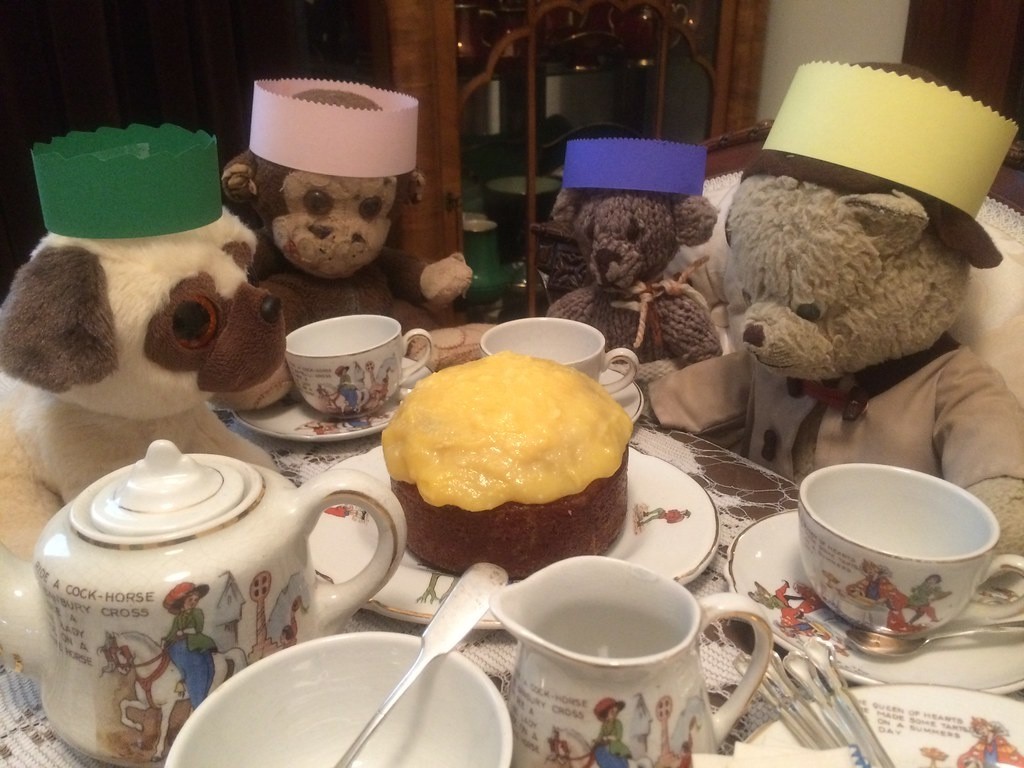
<box><xmin>489</xmin><ymin>555</ymin><xmax>774</xmax><ymax>768</ymax></box>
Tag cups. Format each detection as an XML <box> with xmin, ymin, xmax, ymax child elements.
<box><xmin>799</xmin><ymin>462</ymin><xmax>1024</xmax><ymax>638</ymax></box>
<box><xmin>481</xmin><ymin>174</ymin><xmax>564</xmax><ymax>263</ymax></box>
<box><xmin>283</xmin><ymin>314</ymin><xmax>433</xmax><ymax>420</ymax></box>
<box><xmin>480</xmin><ymin>317</ymin><xmax>639</xmax><ymax>395</ymax></box>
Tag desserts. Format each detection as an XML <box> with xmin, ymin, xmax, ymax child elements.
<box><xmin>379</xmin><ymin>349</ymin><xmax>633</xmax><ymax>581</ymax></box>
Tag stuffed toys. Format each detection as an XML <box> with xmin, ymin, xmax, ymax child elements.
<box><xmin>543</xmin><ymin>184</ymin><xmax>723</xmax><ymax>421</ymax></box>
<box><xmin>0</xmin><ymin>211</ymin><xmax>285</xmax><ymax>565</ymax></box>
<box><xmin>641</xmin><ymin>61</ymin><xmax>1024</xmax><ymax>559</ymax></box>
<box><xmin>215</xmin><ymin>91</ymin><xmax>473</xmax><ymax>416</ymax></box>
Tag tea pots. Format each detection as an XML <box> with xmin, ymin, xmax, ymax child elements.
<box><xmin>0</xmin><ymin>440</ymin><xmax>408</xmax><ymax>768</ymax></box>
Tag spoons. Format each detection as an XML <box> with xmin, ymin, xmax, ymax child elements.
<box><xmin>846</xmin><ymin>620</ymin><xmax>1024</xmax><ymax>658</ymax></box>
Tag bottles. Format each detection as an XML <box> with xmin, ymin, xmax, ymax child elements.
<box><xmin>463</xmin><ymin>219</ymin><xmax>512</xmax><ymax>306</ymax></box>
<box><xmin>455</xmin><ymin>4</ymin><xmax>508</xmax><ymax>143</ymax></box>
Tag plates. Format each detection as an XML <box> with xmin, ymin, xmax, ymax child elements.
<box><xmin>724</xmin><ymin>507</ymin><xmax>1024</xmax><ymax>696</ymax></box>
<box><xmin>599</xmin><ymin>369</ymin><xmax>644</xmax><ymax>425</ymax></box>
<box><xmin>311</xmin><ymin>445</ymin><xmax>720</xmax><ymax>632</ymax></box>
<box><xmin>741</xmin><ymin>683</ymin><xmax>1024</xmax><ymax>768</ymax></box>
<box><xmin>232</xmin><ymin>358</ymin><xmax>432</xmax><ymax>441</ymax></box>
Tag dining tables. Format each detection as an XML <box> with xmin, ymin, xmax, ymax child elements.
<box><xmin>2</xmin><ymin>321</ymin><xmax>1022</xmax><ymax>765</ymax></box>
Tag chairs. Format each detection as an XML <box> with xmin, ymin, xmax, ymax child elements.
<box><xmin>530</xmin><ymin>121</ymin><xmax>1023</xmax><ymax>450</ymax></box>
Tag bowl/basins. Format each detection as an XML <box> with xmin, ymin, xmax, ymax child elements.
<box><xmin>162</xmin><ymin>630</ymin><xmax>514</xmax><ymax>768</ymax></box>
